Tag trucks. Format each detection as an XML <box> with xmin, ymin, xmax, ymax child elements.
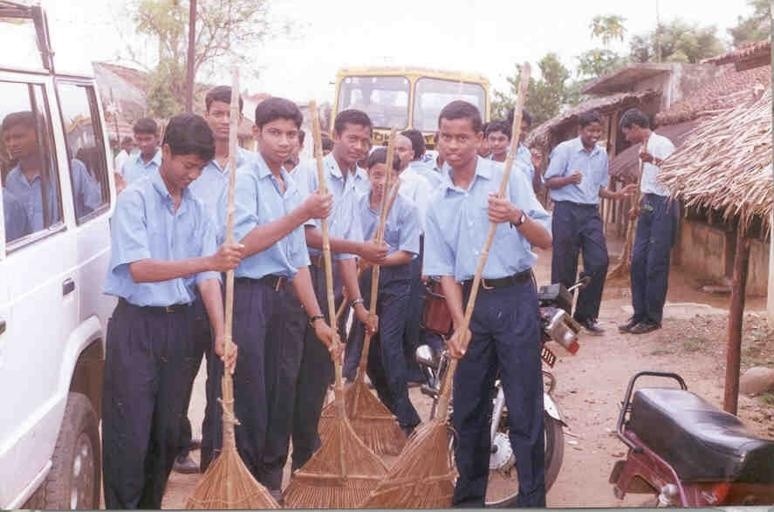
<box><xmin>318</xmin><ymin>68</ymin><xmax>492</xmax><ymax>161</ymax></box>
<box><xmin>0</xmin><ymin>1</ymin><xmax>121</xmax><ymax>511</ymax></box>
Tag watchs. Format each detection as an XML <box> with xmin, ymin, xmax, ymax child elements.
<box><xmin>619</xmin><ymin>108</ymin><xmax>682</xmax><ymax>334</ymax></box>
<box><xmin>510</xmin><ymin>210</ymin><xmax>527</xmax><ymax>228</ymax></box>
<box><xmin>652</xmin><ymin>156</ymin><xmax>656</xmax><ymax>165</ymax></box>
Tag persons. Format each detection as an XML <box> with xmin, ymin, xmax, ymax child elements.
<box><xmin>290</xmin><ymin>109</ymin><xmax>373</xmax><ymax>473</ymax></box>
<box><xmin>102</xmin><ymin>111</ymin><xmax>238</xmax><ymax>510</ymax></box>
<box><xmin>0</xmin><ymin>111</ymin><xmax>103</xmax><ymax>243</ymax></box>
<box><xmin>215</xmin><ymin>97</ymin><xmax>342</xmax><ymax>500</ymax></box>
<box><xmin>358</xmin><ymin>148</ymin><xmax>421</xmax><ymax>437</ymax></box>
<box><xmin>391</xmin><ymin>107</ymin><xmax>554</xmax><ymax>209</ymax></box>
<box><xmin>421</xmin><ymin>100</ymin><xmax>553</xmax><ymax>508</ymax></box>
<box><xmin>105</xmin><ymin>117</ymin><xmax>163</xmax><ymax>193</ymax></box>
<box><xmin>544</xmin><ymin>115</ymin><xmax>637</xmax><ymax>335</ymax></box>
<box><xmin>314</xmin><ymin>138</ymin><xmax>335</xmax><ymax>157</ymax></box>
<box><xmin>172</xmin><ymin>86</ymin><xmax>260</xmax><ymax>472</ymax></box>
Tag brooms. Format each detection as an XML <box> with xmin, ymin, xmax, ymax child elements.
<box><xmin>319</xmin><ymin>127</ymin><xmax>406</xmax><ymax>457</ymax></box>
<box><xmin>358</xmin><ymin>62</ymin><xmax>532</xmax><ymax>509</ymax></box>
<box><xmin>604</xmin><ymin>133</ymin><xmax>649</xmax><ymax>282</ymax></box>
<box><xmin>282</xmin><ymin>101</ymin><xmax>388</xmax><ymax>508</ymax></box>
<box><xmin>187</xmin><ymin>68</ymin><xmax>277</xmax><ymax>510</ymax></box>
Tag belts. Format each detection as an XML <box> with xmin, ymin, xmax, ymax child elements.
<box><xmin>554</xmin><ymin>200</ymin><xmax>600</xmax><ymax>208</ymax></box>
<box><xmin>236</xmin><ymin>273</ymin><xmax>291</xmax><ymax>293</ymax></box>
<box><xmin>461</xmin><ymin>266</ymin><xmax>534</xmax><ymax>292</ymax></box>
<box><xmin>118</xmin><ymin>296</ymin><xmax>203</xmax><ymax>315</ymax></box>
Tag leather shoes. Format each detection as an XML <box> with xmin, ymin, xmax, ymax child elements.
<box><xmin>168</xmin><ymin>439</ymin><xmax>203</xmax><ymax>475</ymax></box>
<box><xmin>618</xmin><ymin>316</ymin><xmax>660</xmax><ymax>333</ymax></box>
<box><xmin>574</xmin><ymin>315</ymin><xmax>605</xmax><ymax>332</ymax></box>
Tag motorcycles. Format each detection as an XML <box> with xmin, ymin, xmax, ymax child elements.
<box><xmin>609</xmin><ymin>369</ymin><xmax>773</xmax><ymax>509</ymax></box>
<box><xmin>366</xmin><ymin>257</ymin><xmax>591</xmax><ymax>510</ymax></box>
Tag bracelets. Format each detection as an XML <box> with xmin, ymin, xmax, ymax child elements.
<box><xmin>307</xmin><ymin>315</ymin><xmax>325</xmax><ymax>328</ymax></box>
<box><xmin>351</xmin><ymin>299</ymin><xmax>363</xmax><ymax>306</ymax></box>
